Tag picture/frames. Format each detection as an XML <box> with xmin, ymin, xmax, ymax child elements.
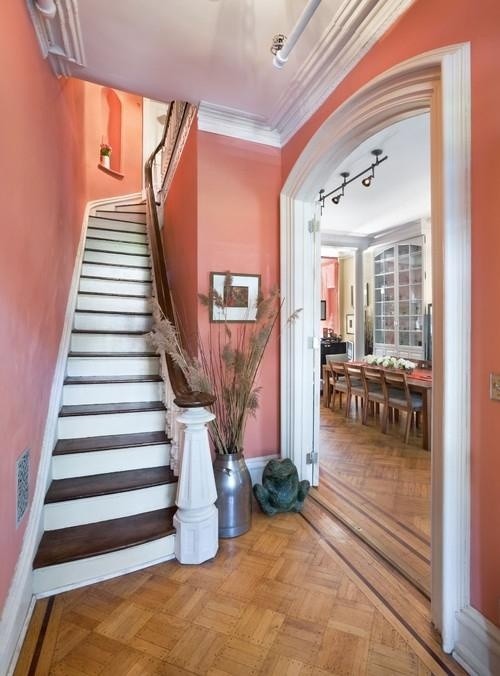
<box><xmin>209</xmin><ymin>270</ymin><xmax>261</xmax><ymax>324</ymax></box>
<box><xmin>345</xmin><ymin>314</ymin><xmax>354</xmax><ymax>334</ymax></box>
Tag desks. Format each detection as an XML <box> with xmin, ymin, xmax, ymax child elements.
<box><xmin>323</xmin><ymin>363</ymin><xmax>431</xmax><ymax>451</ymax></box>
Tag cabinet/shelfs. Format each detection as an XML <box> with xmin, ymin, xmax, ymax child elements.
<box><xmin>368</xmin><ymin>218</ymin><xmax>432</xmax><ymax>360</ymax></box>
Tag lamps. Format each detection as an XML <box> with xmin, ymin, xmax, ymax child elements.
<box><xmin>317</xmin><ymin>149</ymin><xmax>388</xmax><ymax>205</ymax></box>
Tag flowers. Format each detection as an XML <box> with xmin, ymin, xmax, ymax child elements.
<box><xmin>100</xmin><ymin>143</ymin><xmax>113</xmax><ymax>157</ymax></box>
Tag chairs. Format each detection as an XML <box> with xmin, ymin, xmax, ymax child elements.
<box><xmin>325</xmin><ymin>354</ymin><xmax>432</xmax><ymax>444</ymax></box>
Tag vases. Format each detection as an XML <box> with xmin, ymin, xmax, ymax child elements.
<box><xmin>212</xmin><ymin>447</ymin><xmax>252</xmax><ymax>540</ymax></box>
<box><xmin>101</xmin><ymin>155</ymin><xmax>110</xmax><ymax>169</ymax></box>
